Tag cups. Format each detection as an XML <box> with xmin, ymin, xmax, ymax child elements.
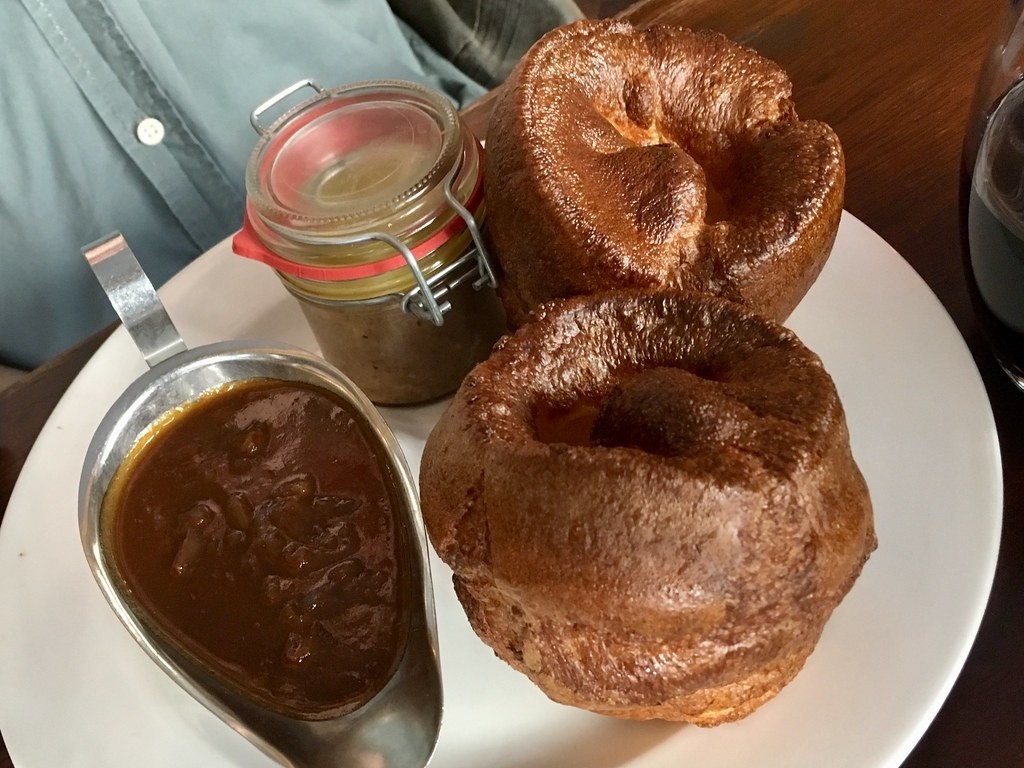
<box><xmin>72</xmin><ymin>230</ymin><xmax>445</xmax><ymax>768</ymax></box>
<box><xmin>236</xmin><ymin>70</ymin><xmax>504</xmax><ymax>413</ymax></box>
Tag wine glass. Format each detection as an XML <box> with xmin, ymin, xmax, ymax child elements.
<box><xmin>956</xmin><ymin>3</ymin><xmax>1024</xmax><ymax>394</ymax></box>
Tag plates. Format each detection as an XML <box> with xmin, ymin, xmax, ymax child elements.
<box><xmin>0</xmin><ymin>205</ymin><xmax>1010</xmax><ymax>768</ymax></box>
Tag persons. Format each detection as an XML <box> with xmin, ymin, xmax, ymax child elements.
<box><xmin>0</xmin><ymin>0</ymin><xmax>584</xmax><ymax>391</ymax></box>
